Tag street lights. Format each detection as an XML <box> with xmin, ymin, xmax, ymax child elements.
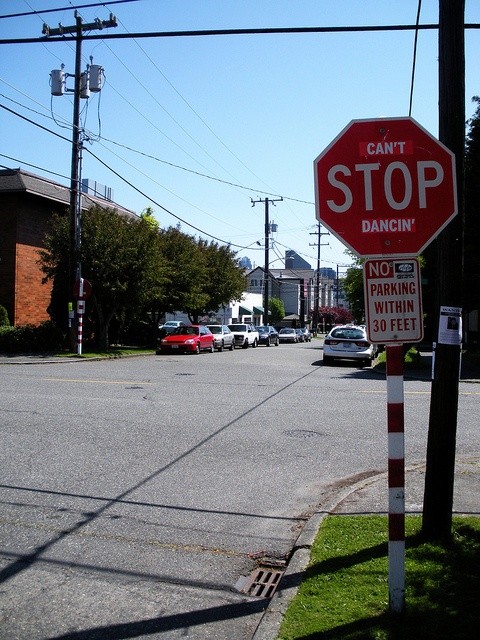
<box><xmin>260</xmin><ymin>256</ymin><xmax>295</xmax><ymax>294</ymax></box>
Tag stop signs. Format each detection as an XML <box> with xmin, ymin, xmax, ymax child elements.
<box><xmin>313</xmin><ymin>117</ymin><xmax>458</xmax><ymax>259</ymax></box>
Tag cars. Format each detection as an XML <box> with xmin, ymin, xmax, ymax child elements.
<box><xmin>255</xmin><ymin>326</ymin><xmax>280</xmax><ymax>346</ymax></box>
<box><xmin>278</xmin><ymin>328</ymin><xmax>298</xmax><ymax>343</ymax></box>
<box><xmin>323</xmin><ymin>325</ymin><xmax>379</xmax><ymax>367</ymax></box>
<box><xmin>228</xmin><ymin>324</ymin><xmax>259</xmax><ymax>349</ymax></box>
<box><xmin>206</xmin><ymin>325</ymin><xmax>235</xmax><ymax>352</ymax></box>
<box><xmin>160</xmin><ymin>324</ymin><xmax>215</xmax><ymax>355</ymax></box>
<box><xmin>159</xmin><ymin>321</ymin><xmax>185</xmax><ymax>332</ymax></box>
<box><xmin>301</xmin><ymin>328</ymin><xmax>313</xmax><ymax>342</ymax></box>
<box><xmin>296</xmin><ymin>329</ymin><xmax>306</xmax><ymax>343</ymax></box>
